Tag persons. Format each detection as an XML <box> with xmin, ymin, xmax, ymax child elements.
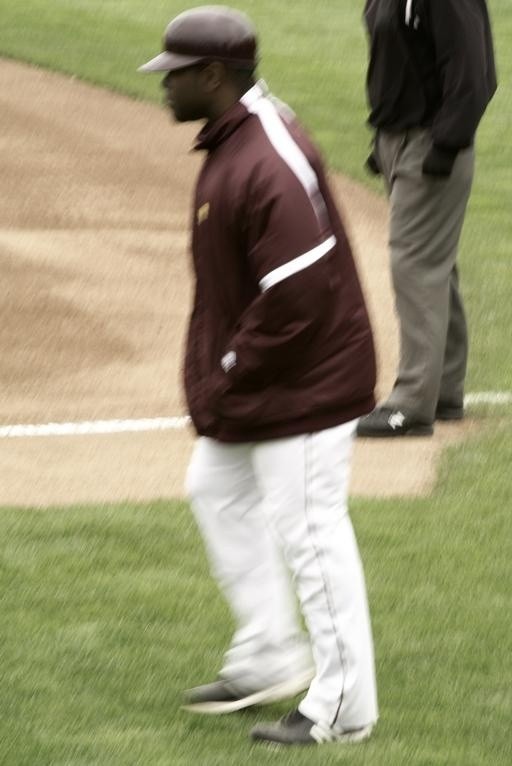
<box><xmin>133</xmin><ymin>6</ymin><xmax>378</xmax><ymax>748</ymax></box>
<box><xmin>353</xmin><ymin>0</ymin><xmax>499</xmax><ymax>438</ymax></box>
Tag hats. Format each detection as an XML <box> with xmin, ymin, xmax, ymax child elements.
<box><xmin>136</xmin><ymin>5</ymin><xmax>259</xmax><ymax>73</ymax></box>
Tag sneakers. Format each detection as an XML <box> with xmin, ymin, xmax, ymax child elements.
<box><xmin>178</xmin><ymin>642</ymin><xmax>314</xmax><ymax>713</ymax></box>
<box><xmin>250</xmin><ymin>719</ymin><xmax>373</xmax><ymax>746</ymax></box>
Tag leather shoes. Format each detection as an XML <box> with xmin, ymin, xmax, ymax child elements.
<box><xmin>358</xmin><ymin>399</ymin><xmax>464</xmax><ymax>439</ymax></box>
